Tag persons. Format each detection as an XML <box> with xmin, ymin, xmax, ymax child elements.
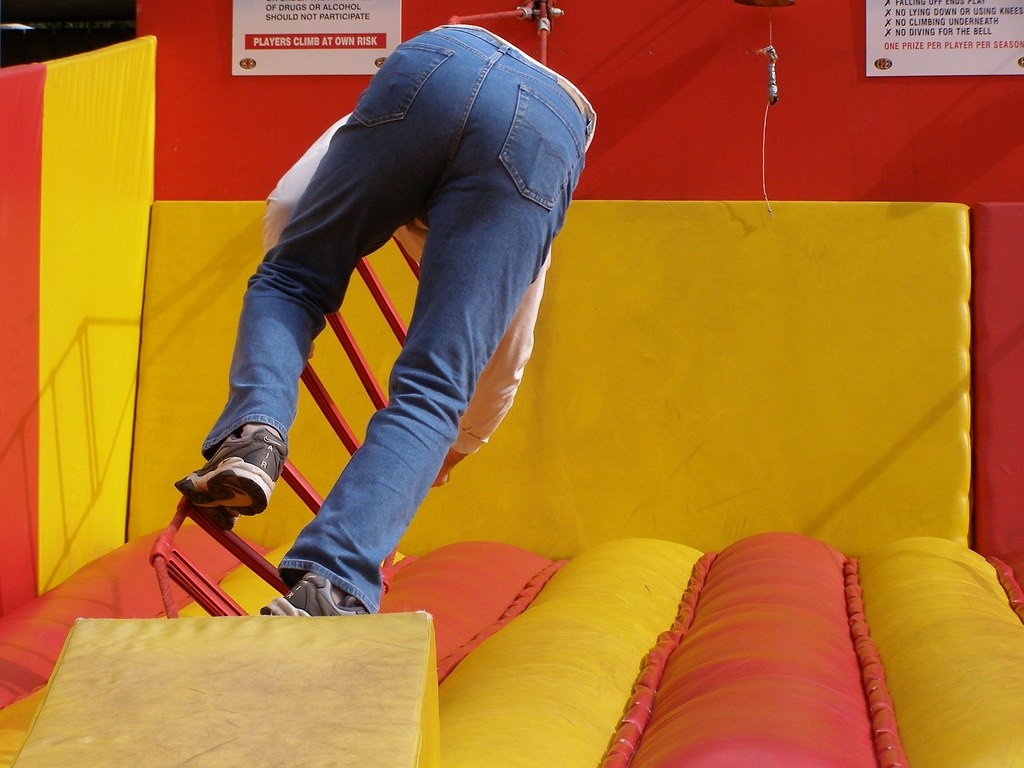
<box><xmin>174</xmin><ymin>23</ymin><xmax>596</xmax><ymax>615</ymax></box>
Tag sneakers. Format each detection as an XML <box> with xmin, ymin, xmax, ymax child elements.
<box><xmin>172</xmin><ymin>428</ymin><xmax>288</xmax><ymax>528</ymax></box>
<box><xmin>260</xmin><ymin>572</ymin><xmax>369</xmax><ymax>616</ymax></box>
